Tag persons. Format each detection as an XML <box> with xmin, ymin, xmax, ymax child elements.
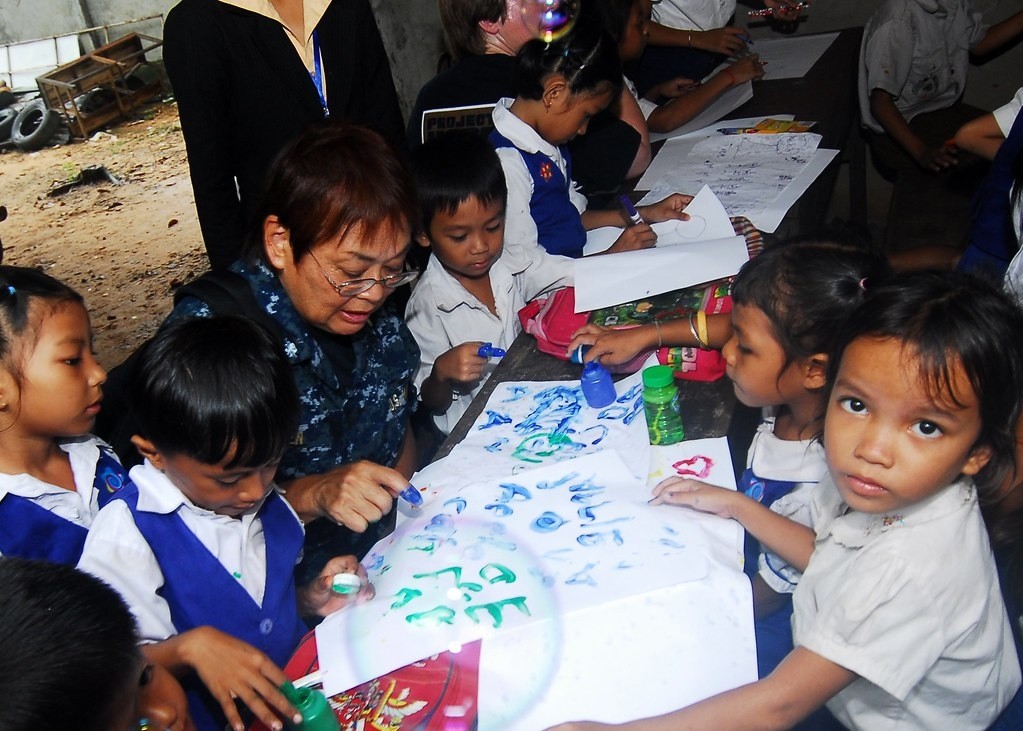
<box><xmin>1</xmin><ymin>0</ymin><xmax>1023</xmax><ymax>730</ymax></box>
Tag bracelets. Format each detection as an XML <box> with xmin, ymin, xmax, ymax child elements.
<box><xmin>688</xmin><ymin>29</ymin><xmax>690</xmax><ymax>46</ymax></box>
<box><xmin>689</xmin><ymin>312</ymin><xmax>708</xmax><ymax>350</ymax></box>
<box><xmin>655</xmin><ymin>321</ymin><xmax>661</xmax><ymax>347</ymax></box>
<box><xmin>720</xmin><ymin>69</ymin><xmax>735</xmax><ymax>84</ymax></box>
<box><xmin>697</xmin><ymin>311</ymin><xmax>707</xmax><ymax>347</ymax></box>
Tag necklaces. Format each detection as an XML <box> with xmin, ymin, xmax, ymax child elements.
<box><xmin>308</xmin><ymin>30</ymin><xmax>329</xmax><ymax>116</ymax></box>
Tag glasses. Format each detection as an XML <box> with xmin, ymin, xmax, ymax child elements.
<box><xmin>308</xmin><ymin>249</ymin><xmax>420</xmax><ymax>297</ymax></box>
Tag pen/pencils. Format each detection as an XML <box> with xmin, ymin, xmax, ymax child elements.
<box><xmin>716</xmin><ymin>128</ymin><xmax>759</xmax><ymax>135</ymax></box>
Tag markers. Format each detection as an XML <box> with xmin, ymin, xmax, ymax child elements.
<box><xmin>735</xmin><ymin>34</ymin><xmax>753</xmax><ymax>45</ymax></box>
<box><xmin>758</xmin><ymin>61</ymin><xmax>769</xmax><ymax>65</ymax></box>
<box><xmin>619</xmin><ymin>194</ymin><xmax>644</xmax><ymax>224</ymax></box>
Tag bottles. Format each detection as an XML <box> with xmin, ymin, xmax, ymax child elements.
<box><xmin>641</xmin><ymin>365</ymin><xmax>685</xmax><ymax>445</ymax></box>
<box><xmin>580</xmin><ymin>361</ymin><xmax>617</xmax><ymax>408</ymax></box>
<box><xmin>285</xmin><ymin>686</ymin><xmax>342</xmax><ymax>731</ymax></box>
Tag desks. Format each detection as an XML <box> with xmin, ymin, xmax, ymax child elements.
<box><xmin>431</xmin><ymin>24</ymin><xmax>867</xmax><ymax>462</ymax></box>
<box><xmin>36</xmin><ymin>32</ymin><xmax>163</xmax><ymax>141</ymax></box>
